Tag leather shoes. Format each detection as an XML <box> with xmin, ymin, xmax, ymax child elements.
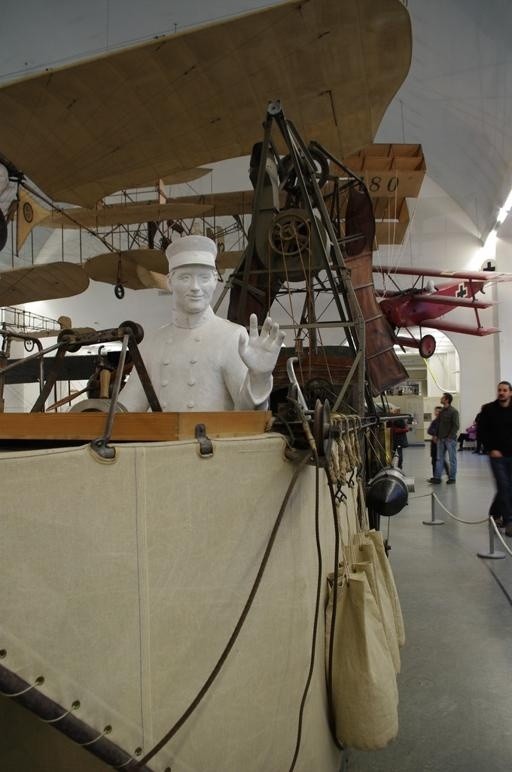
<box><xmin>494</xmin><ymin>518</ymin><xmax>503</xmax><ymax>528</ymax></box>
<box><xmin>429</xmin><ymin>478</ymin><xmax>441</xmax><ymax>484</ymax></box>
<box><xmin>447</xmin><ymin>479</ymin><xmax>456</xmax><ymax>484</ymax></box>
<box><xmin>505</xmin><ymin>522</ymin><xmax>512</xmax><ymax>537</ymax></box>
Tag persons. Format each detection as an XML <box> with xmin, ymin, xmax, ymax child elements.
<box><xmin>427</xmin><ymin>394</ymin><xmax>460</xmax><ymax>485</ymax></box>
<box><xmin>456</xmin><ymin>413</ymin><xmax>488</xmax><ymax>455</ymax></box>
<box><xmin>428</xmin><ymin>407</ymin><xmax>449</xmax><ymax>478</ymax></box>
<box><xmin>114</xmin><ymin>234</ymin><xmax>286</xmax><ymax>412</ymax></box>
<box><xmin>386</xmin><ymin>408</ymin><xmax>409</xmax><ymax>468</ymax></box>
<box><xmin>480</xmin><ymin>382</ymin><xmax>512</xmax><ymax>538</ymax></box>
